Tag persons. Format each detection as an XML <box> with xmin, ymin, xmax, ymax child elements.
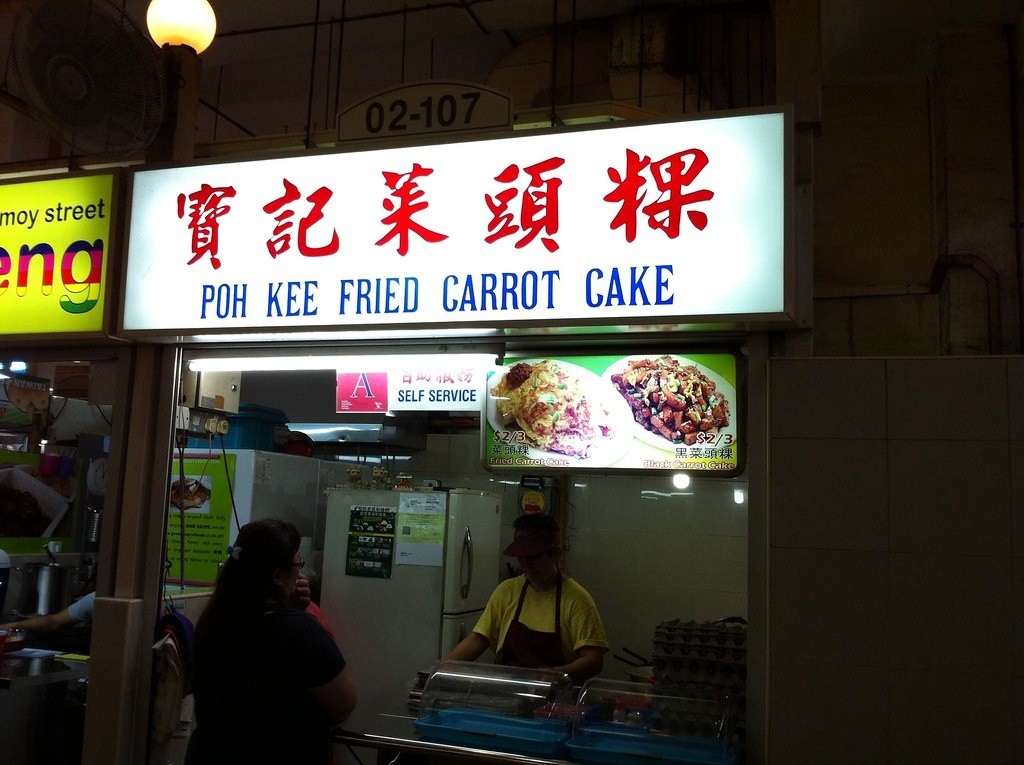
<box><xmin>189</xmin><ymin>519</ymin><xmax>358</xmax><ymax>765</ymax></box>
<box><xmin>433</xmin><ymin>511</ymin><xmax>609</xmax><ymax>684</ymax></box>
<box><xmin>0</xmin><ymin>592</ymin><xmax>95</xmax><ymax>631</ymax></box>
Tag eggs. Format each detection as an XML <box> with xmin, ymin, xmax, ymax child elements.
<box><xmin>613</xmin><ymin>709</ymin><xmax>644</xmax><ymax>724</ymax></box>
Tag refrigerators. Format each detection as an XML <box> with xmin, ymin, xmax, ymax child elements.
<box><xmin>321</xmin><ymin>489</ymin><xmax>501</xmax><ymax>765</ymax></box>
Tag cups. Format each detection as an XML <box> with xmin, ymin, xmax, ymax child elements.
<box><xmin>39</xmin><ymin>453</ymin><xmax>58</xmax><ymax>477</ymax></box>
<box><xmin>57</xmin><ymin>456</ymin><xmax>75</xmax><ymax>476</ymax></box>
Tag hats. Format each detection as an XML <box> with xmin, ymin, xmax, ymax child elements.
<box><xmin>503</xmin><ymin>527</ymin><xmax>559</xmax><ymax>557</ymax></box>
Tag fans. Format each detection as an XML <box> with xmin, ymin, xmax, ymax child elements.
<box><xmin>11</xmin><ymin>0</ymin><xmax>181</xmax><ymax>162</ymax></box>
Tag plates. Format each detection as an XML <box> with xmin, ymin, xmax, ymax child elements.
<box><xmin>487</xmin><ymin>359</ymin><xmax>634</xmax><ymax>467</ymax></box>
<box><xmin>602</xmin><ymin>355</ymin><xmax>737</xmax><ymax>452</ymax></box>
<box><xmin>87</xmin><ymin>458</ymin><xmax>107</xmax><ymax>496</ymax></box>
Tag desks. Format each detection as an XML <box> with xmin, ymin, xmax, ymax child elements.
<box><xmin>335</xmin><ymin>699</ymin><xmax>581</xmax><ymax>765</ymax></box>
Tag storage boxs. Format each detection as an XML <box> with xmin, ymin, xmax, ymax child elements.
<box><xmin>186</xmin><ymin>402</ymin><xmax>290</xmax><ymax>452</ymax></box>
<box><xmin>0</xmin><ymin>463</ymin><xmax>77</xmax><ymax>538</ymax></box>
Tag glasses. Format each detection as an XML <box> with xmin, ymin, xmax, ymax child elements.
<box><xmin>293</xmin><ymin>558</ymin><xmax>305</xmax><ymax>569</ymax></box>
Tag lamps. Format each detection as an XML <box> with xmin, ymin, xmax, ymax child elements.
<box><xmin>147</xmin><ymin>0</ymin><xmax>218</xmax><ymax>54</ymax></box>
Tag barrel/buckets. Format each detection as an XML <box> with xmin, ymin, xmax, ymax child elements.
<box><xmin>14</xmin><ymin>563</ymin><xmax>65</xmax><ymax>617</ymax></box>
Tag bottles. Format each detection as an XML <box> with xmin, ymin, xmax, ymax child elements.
<box><xmin>613</xmin><ymin>702</ymin><xmax>626</xmax><ymax>722</ymax></box>
